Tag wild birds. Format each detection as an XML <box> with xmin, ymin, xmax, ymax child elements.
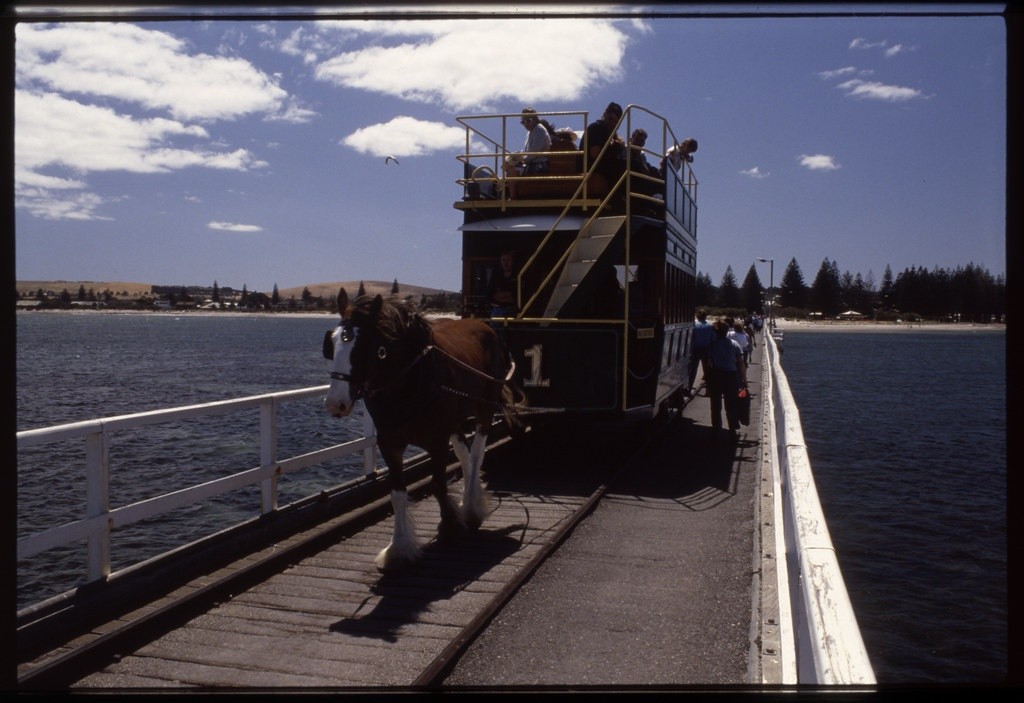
<box><xmin>385</xmin><ymin>156</ymin><xmax>400</xmax><ymax>166</ymax></box>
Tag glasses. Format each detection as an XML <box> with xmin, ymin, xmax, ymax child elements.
<box><xmin>520</xmin><ymin>117</ymin><xmax>533</xmax><ymax>124</ymax></box>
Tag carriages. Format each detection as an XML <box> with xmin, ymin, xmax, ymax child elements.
<box><xmin>325</xmin><ymin>104</ymin><xmax>699</xmax><ymax>570</ymax></box>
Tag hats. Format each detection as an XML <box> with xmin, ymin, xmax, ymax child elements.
<box><xmin>555</xmin><ymin>126</ymin><xmax>578</xmax><ymax>141</ymax></box>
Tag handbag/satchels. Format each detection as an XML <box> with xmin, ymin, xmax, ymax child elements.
<box><xmin>730</xmin><ymin>384</ymin><xmax>751</xmax><ymax>427</ymax></box>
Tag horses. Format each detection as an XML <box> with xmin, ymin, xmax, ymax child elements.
<box><xmin>323</xmin><ymin>287</ymin><xmax>535</xmax><ymax>576</ymax></box>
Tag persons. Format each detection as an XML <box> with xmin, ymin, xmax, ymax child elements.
<box><xmin>660</xmin><ymin>138</ymin><xmax>698</xmax><ymax>175</ymax></box>
<box><xmin>497</xmin><ymin>108</ymin><xmax>578</xmax><ymax>200</ymax></box>
<box><xmin>576</xmin><ymin>102</ymin><xmax>663</xmax><ymax>199</ymax></box>
<box><xmin>684</xmin><ymin>309</ymin><xmax>764</xmax><ymax>438</ymax></box>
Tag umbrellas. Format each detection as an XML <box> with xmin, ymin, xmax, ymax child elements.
<box><xmin>840</xmin><ymin>310</ymin><xmax>861</xmax><ymax>317</ymax></box>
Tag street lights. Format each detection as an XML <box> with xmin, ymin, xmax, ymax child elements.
<box><xmin>757</xmin><ymin>256</ymin><xmax>774</xmax><ymax>334</ymax></box>
